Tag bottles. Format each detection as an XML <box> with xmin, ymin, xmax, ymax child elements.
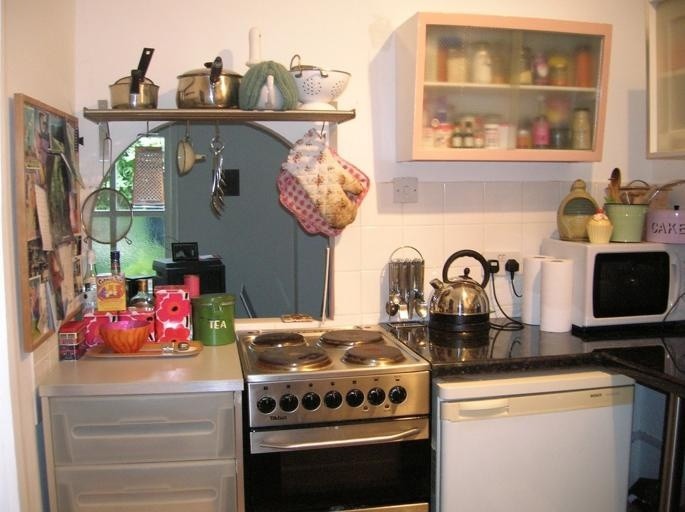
<box><xmin>108</xmin><ymin>247</ymin><xmax>121</xmax><ymax>274</ymax></box>
<box><xmin>584</xmin><ymin>205</ymin><xmax>614</xmax><ymax>244</ymax></box>
<box><xmin>83</xmin><ymin>249</ymin><xmax>99</xmax><ymax>313</ymax></box>
<box><xmin>422</xmin><ymin>33</ymin><xmax>598</xmax><ymax>152</ymax></box>
<box><xmin>128</xmin><ymin>279</ymin><xmax>153</xmax><ymax>338</ymax></box>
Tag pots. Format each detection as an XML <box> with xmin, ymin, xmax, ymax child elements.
<box><xmin>109</xmin><ymin>45</ymin><xmax>161</xmax><ymax>107</ymax></box>
<box><xmin>176</xmin><ymin>54</ymin><xmax>242</xmax><ymax>107</ymax></box>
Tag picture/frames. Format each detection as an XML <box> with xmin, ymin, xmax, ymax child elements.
<box><xmin>14</xmin><ymin>92</ymin><xmax>86</xmax><ymax>353</ymax></box>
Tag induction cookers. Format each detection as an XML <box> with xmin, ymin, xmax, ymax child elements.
<box><xmin>234</xmin><ymin>325</ymin><xmax>430</xmax><ymax>429</ymax></box>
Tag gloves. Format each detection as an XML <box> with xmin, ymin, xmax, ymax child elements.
<box><xmin>282</xmin><ymin>128</ymin><xmax>363</xmax><ymax>229</ymax></box>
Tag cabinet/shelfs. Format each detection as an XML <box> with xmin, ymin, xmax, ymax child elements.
<box><xmin>393</xmin><ymin>12</ymin><xmax>614</xmax><ymax>163</ymax></box>
<box><xmin>643</xmin><ymin>0</ymin><xmax>684</xmax><ymax>161</ymax></box>
<box><xmin>38</xmin><ymin>339</ymin><xmax>246</xmax><ymax>512</ymax></box>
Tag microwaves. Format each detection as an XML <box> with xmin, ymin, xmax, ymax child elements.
<box><xmin>543</xmin><ymin>240</ymin><xmax>684</xmax><ymax>336</ymax></box>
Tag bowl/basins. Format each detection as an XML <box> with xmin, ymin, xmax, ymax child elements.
<box><xmin>602</xmin><ymin>202</ymin><xmax>650</xmax><ymax>243</ymax></box>
<box><xmin>97</xmin><ymin>319</ymin><xmax>151</xmax><ymax>354</ymax></box>
<box><xmin>646</xmin><ymin>208</ymin><xmax>684</xmax><ymax>243</ymax></box>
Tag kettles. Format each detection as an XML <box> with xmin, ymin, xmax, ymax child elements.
<box><xmin>428</xmin><ymin>250</ymin><xmax>496</xmax><ymax>333</ymax></box>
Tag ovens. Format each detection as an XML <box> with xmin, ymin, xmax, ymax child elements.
<box><xmin>243</xmin><ymin>414</ymin><xmax>435</xmax><ymax>511</ymax></box>
